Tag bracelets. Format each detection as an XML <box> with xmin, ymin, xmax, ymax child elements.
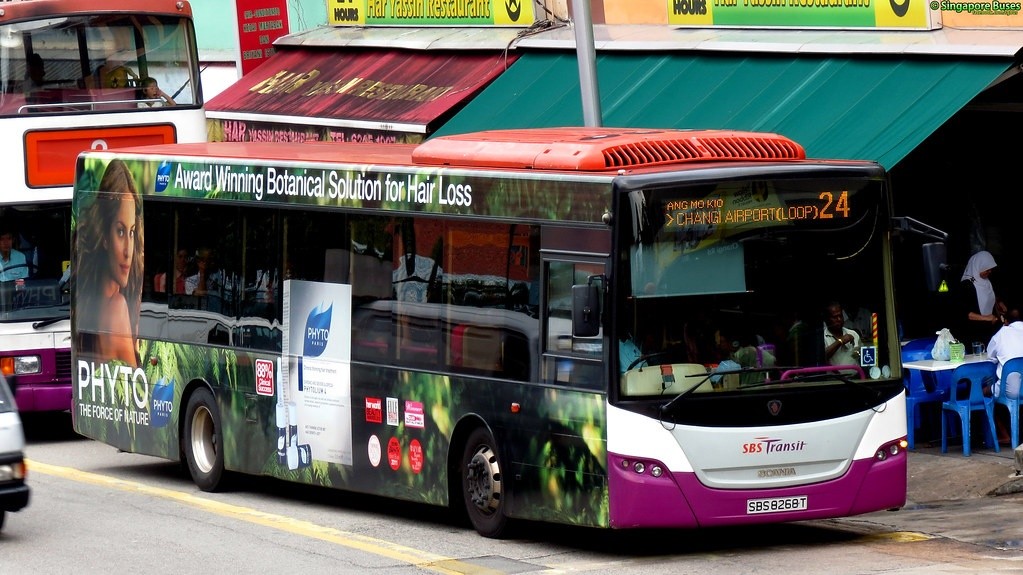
<box><xmin>838</xmin><ymin>339</ymin><xmax>843</xmax><ymax>345</ymax></box>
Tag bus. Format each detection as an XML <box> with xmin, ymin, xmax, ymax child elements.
<box><xmin>65</xmin><ymin>125</ymin><xmax>948</xmax><ymax>540</ymax></box>
<box><xmin>0</xmin><ymin>0</ymin><xmax>205</xmax><ymax>412</ymax></box>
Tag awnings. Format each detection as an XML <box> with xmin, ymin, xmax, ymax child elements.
<box><xmin>203</xmin><ymin>46</ymin><xmax>523</xmax><ymax>143</ymax></box>
<box><xmin>423</xmin><ymin>51</ymin><xmax>1015</xmax><ymax>176</ymax></box>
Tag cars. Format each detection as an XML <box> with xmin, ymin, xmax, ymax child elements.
<box><xmin>0</xmin><ymin>372</ymin><xmax>31</xmax><ymax>533</ymax></box>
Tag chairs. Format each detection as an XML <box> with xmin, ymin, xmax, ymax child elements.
<box><xmin>899</xmin><ymin>341</ymin><xmax>1023</xmax><ymax>456</ymax></box>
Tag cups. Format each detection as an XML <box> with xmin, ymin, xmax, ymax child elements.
<box><xmin>972</xmin><ymin>342</ymin><xmax>985</xmax><ymax>358</ymax></box>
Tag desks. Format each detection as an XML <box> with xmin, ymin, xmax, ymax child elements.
<box><xmin>901</xmin><ymin>353</ymin><xmax>1000</xmax><ymax>438</ymax></box>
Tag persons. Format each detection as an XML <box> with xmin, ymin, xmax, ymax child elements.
<box><xmin>823</xmin><ymin>304</ymin><xmax>866</xmax><ymax>379</ymax></box>
<box><xmin>73</xmin><ymin>161</ymin><xmax>148</xmax><ymax>369</ymax></box>
<box><xmin>106</xmin><ymin>66</ymin><xmax>137</xmax><ymax>89</ymax></box>
<box><xmin>135</xmin><ymin>77</ymin><xmax>176</xmax><ymax>106</ymax></box>
<box><xmin>618</xmin><ymin>317</ymin><xmax>648</xmax><ymax>375</ymax></box>
<box><xmin>450</xmin><ymin>289</ymin><xmax>482</xmax><ymax>369</ymax></box>
<box><xmin>953</xmin><ymin>251</ymin><xmax>1008</xmax><ymax>352</ymax></box>
<box><xmin>159</xmin><ymin>240</ymin><xmax>218</xmax><ymax>297</ymax></box>
<box><xmin>982</xmin><ymin>306</ymin><xmax>1023</xmax><ymax>445</ymax></box>
<box><xmin>18</xmin><ymin>52</ymin><xmax>50</xmax><ymax>93</ymax></box>
<box><xmin>0</xmin><ymin>228</ymin><xmax>30</xmax><ymax>282</ymax></box>
